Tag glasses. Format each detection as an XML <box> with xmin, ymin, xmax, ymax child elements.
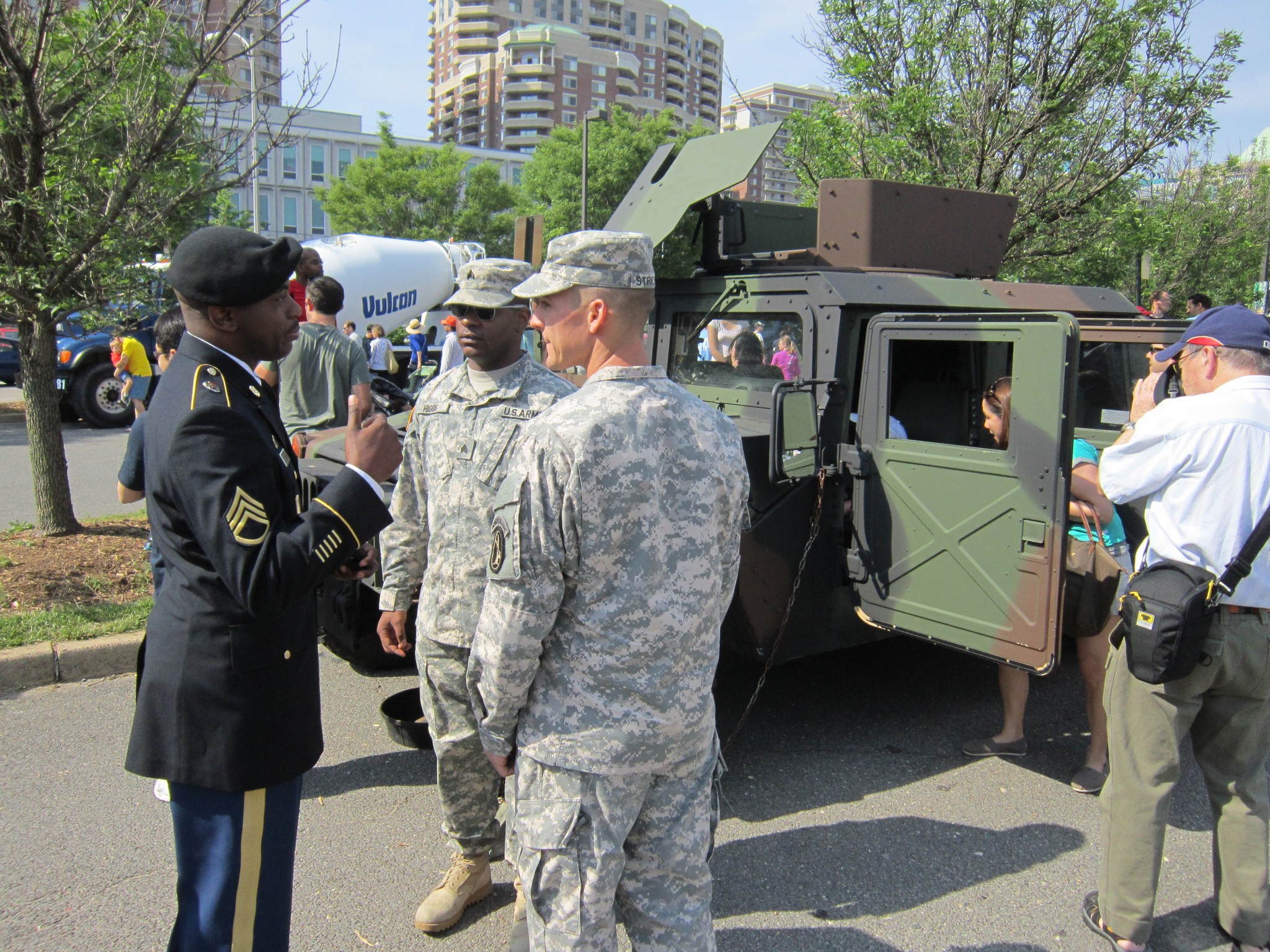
<box><xmin>777</xmin><ymin>342</ymin><xmax>785</xmax><ymax>345</ymax></box>
<box><xmin>153</xmin><ymin>351</ymin><xmax>166</xmax><ymax>360</ymax></box>
<box><xmin>1173</xmin><ymin>348</ymin><xmax>1203</xmax><ymax>375</ymax></box>
<box><xmin>300</xmin><ymin>261</ymin><xmax>324</xmax><ymax>264</ymax></box>
<box><xmin>1149</xmin><ymin>345</ymin><xmax>1164</xmax><ymax>356</ymax></box>
<box><xmin>759</xmin><ymin>325</ymin><xmax>763</xmax><ymax>327</ymax></box>
<box><xmin>371</xmin><ymin>331</ymin><xmax>373</xmax><ymax>333</ymax></box>
<box><xmin>443</xmin><ymin>324</ymin><xmax>448</xmax><ymax>326</ymax></box>
<box><xmin>343</xmin><ymin>326</ymin><xmax>349</xmax><ymax>330</ymax></box>
<box><xmin>450</xmin><ymin>304</ymin><xmax>528</xmax><ymax>320</ymax></box>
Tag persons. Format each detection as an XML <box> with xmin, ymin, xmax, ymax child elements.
<box><xmin>1136</xmin><ymin>306</ymin><xmax>1152</xmax><ymax>319</ymax></box>
<box><xmin>1185</xmin><ymin>293</ymin><xmax>1211</xmax><ymax>321</ymax></box>
<box><xmin>253</xmin><ymin>275</ymin><xmax>371</xmax><ymax>449</ymax></box>
<box><xmin>374</xmin><ymin>258</ymin><xmax>577</xmax><ymax>937</ymax></box>
<box><xmin>286</xmin><ymin>248</ymin><xmax>337</xmax><ymax>329</ymax></box>
<box><xmin>464</xmin><ymin>229</ymin><xmax>749</xmax><ymax>952</ymax></box>
<box><xmin>1080</xmin><ymin>302</ymin><xmax>1270</xmax><ymax>952</ymax></box>
<box><xmin>345</xmin><ymin>306</ymin><xmax>801</xmax><ymax>389</ymax></box>
<box><xmin>123</xmin><ymin>227</ymin><xmax>406</xmax><ymax>952</ymax></box>
<box><xmin>112</xmin><ymin>328</ymin><xmax>153</xmax><ymax>432</ymax></box>
<box><xmin>151</xmin><ymin>307</ymin><xmax>188</xmax><ymax>801</ymax></box>
<box><xmin>1146</xmin><ymin>291</ymin><xmax>1177</xmax><ymax>327</ymax></box>
<box><xmin>842</xmin><ymin>413</ymin><xmax>907</xmax><ymax>516</ymax></box>
<box><xmin>109</xmin><ymin>339</ymin><xmax>132</xmax><ymax>409</ymax></box>
<box><xmin>1144</xmin><ymin>341</ymin><xmax>1180</xmax><ymax>377</ymax></box>
<box><xmin>961</xmin><ymin>377</ymin><xmax>1133</xmax><ymax>793</ymax></box>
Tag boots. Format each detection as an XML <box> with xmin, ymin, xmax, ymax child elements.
<box><xmin>413</xmin><ymin>851</ymin><xmax>492</xmax><ymax>932</ymax></box>
<box><xmin>509</xmin><ymin>877</ymin><xmax>530</xmax><ymax>952</ymax></box>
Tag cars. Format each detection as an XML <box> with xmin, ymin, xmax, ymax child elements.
<box><xmin>288</xmin><ymin>125</ymin><xmax>1199</xmax><ymax>682</ymax></box>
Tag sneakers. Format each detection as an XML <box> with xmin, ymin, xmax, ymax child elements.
<box><xmin>153</xmin><ymin>779</ymin><xmax>170</xmax><ymax>803</ymax></box>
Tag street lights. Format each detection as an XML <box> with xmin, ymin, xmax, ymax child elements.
<box><xmin>197</xmin><ymin>27</ymin><xmax>262</xmax><ymax>234</ymax></box>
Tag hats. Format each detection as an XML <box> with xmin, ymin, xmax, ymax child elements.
<box><xmin>406</xmin><ymin>319</ymin><xmax>425</xmax><ymax>334</ymax></box>
<box><xmin>444</xmin><ymin>258</ymin><xmax>531</xmax><ymax>308</ymax></box>
<box><xmin>1136</xmin><ymin>306</ymin><xmax>1153</xmax><ymax>315</ymax></box>
<box><xmin>510</xmin><ymin>230</ymin><xmax>654</xmax><ymax>297</ymax></box>
<box><xmin>440</xmin><ymin>316</ymin><xmax>457</xmax><ymax>327</ymax></box>
<box><xmin>1156</xmin><ymin>304</ymin><xmax>1269</xmax><ymax>362</ymax></box>
<box><xmin>166</xmin><ymin>226</ymin><xmax>304</xmax><ymax>308</ymax></box>
<box><xmin>754</xmin><ymin>322</ymin><xmax>765</xmax><ymax>326</ymax></box>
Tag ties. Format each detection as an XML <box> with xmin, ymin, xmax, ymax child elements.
<box><xmin>256</xmin><ymin>376</ymin><xmax>281</xmax><ymax>419</ymax></box>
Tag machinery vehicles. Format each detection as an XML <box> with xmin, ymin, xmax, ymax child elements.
<box><xmin>15</xmin><ymin>234</ymin><xmax>488</xmax><ymax>424</ymax></box>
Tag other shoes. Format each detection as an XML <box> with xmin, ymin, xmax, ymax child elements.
<box><xmin>1071</xmin><ymin>760</ymin><xmax>1113</xmax><ymax>792</ymax></box>
<box><xmin>962</xmin><ymin>736</ymin><xmax>1027</xmax><ymax>756</ymax></box>
<box><xmin>123</xmin><ymin>428</ymin><xmax>130</xmax><ymax>432</ymax></box>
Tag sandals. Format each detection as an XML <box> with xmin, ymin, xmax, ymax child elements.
<box><xmin>1216</xmin><ymin>912</ymin><xmax>1270</xmax><ymax>952</ymax></box>
<box><xmin>1081</xmin><ymin>891</ymin><xmax>1152</xmax><ymax>952</ymax></box>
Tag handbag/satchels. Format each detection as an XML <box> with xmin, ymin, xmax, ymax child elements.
<box><xmin>1108</xmin><ymin>561</ymin><xmax>1217</xmax><ymax>685</ymax></box>
<box><xmin>1063</xmin><ymin>499</ymin><xmax>1129</xmax><ymax>637</ymax></box>
<box><xmin>386</xmin><ymin>339</ymin><xmax>399</xmax><ymax>374</ymax></box>
<box><xmin>407</xmin><ymin>363</ymin><xmax>417</xmax><ymax>370</ymax></box>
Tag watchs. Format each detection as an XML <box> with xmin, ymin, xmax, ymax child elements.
<box><xmin>1120</xmin><ymin>421</ymin><xmax>1135</xmax><ymax>433</ymax></box>
